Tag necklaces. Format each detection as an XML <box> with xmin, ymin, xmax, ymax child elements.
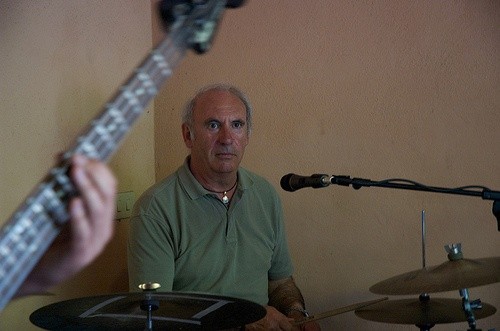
<box><xmin>195</xmin><ymin>176</ymin><xmax>238</xmax><ymax>205</ymax></box>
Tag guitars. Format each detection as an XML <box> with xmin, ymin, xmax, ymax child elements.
<box><xmin>0</xmin><ymin>0</ymin><xmax>247</xmax><ymax>316</ymax></box>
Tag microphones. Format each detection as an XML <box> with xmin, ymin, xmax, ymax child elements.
<box><xmin>280</xmin><ymin>173</ymin><xmax>333</xmax><ymax>192</ymax></box>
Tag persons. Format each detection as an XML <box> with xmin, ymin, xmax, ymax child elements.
<box><xmin>13</xmin><ymin>151</ymin><xmax>117</xmax><ymax>301</ymax></box>
<box><xmin>127</xmin><ymin>84</ymin><xmax>322</xmax><ymax>331</ymax></box>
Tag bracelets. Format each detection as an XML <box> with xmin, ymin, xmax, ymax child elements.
<box><xmin>285</xmin><ymin>304</ymin><xmax>310</xmax><ymax>318</ymax></box>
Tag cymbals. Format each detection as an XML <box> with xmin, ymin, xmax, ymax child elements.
<box><xmin>354</xmin><ymin>297</ymin><xmax>498</xmax><ymax>324</ymax></box>
<box><xmin>369</xmin><ymin>256</ymin><xmax>500</xmax><ymax>296</ymax></box>
<box><xmin>29</xmin><ymin>291</ymin><xmax>269</xmax><ymax>331</ymax></box>
